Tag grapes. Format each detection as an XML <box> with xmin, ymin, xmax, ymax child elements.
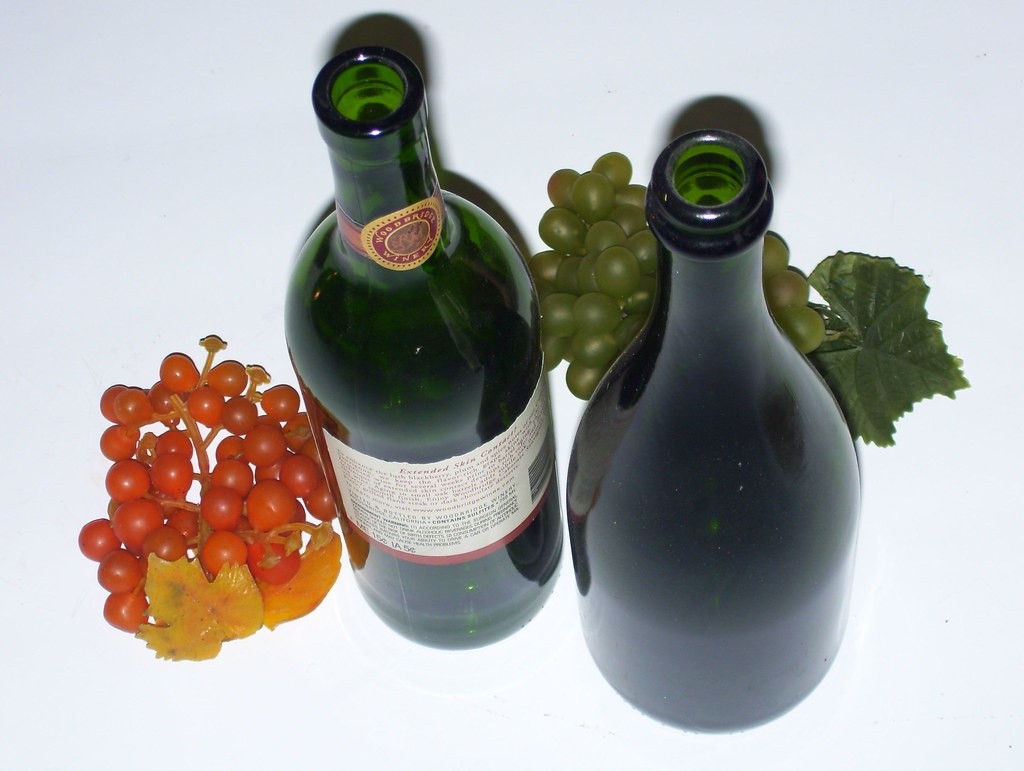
<box><xmin>527</xmin><ymin>151</ymin><xmax>828</xmax><ymax>401</ymax></box>
<box><xmin>77</xmin><ymin>352</ymin><xmax>338</xmax><ymax>632</ymax></box>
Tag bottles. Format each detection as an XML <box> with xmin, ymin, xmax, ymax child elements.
<box><xmin>567</xmin><ymin>128</ymin><xmax>859</xmax><ymax>738</ymax></box>
<box><xmin>281</xmin><ymin>49</ymin><xmax>566</xmax><ymax>650</ymax></box>
